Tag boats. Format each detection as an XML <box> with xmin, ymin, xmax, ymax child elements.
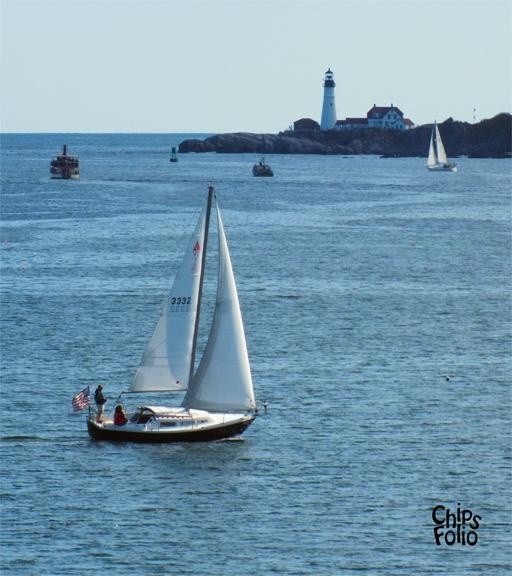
<box><xmin>251</xmin><ymin>159</ymin><xmax>276</xmax><ymax>176</ymax></box>
<box><xmin>46</xmin><ymin>141</ymin><xmax>81</xmax><ymax>180</ymax></box>
<box><xmin>168</xmin><ymin>145</ymin><xmax>179</xmax><ymax>162</ymax></box>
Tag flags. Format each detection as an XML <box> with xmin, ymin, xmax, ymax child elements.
<box><xmin>71</xmin><ymin>385</ymin><xmax>91</xmax><ymax>411</ymax></box>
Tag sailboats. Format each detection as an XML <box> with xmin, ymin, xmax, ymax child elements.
<box><xmin>424</xmin><ymin>117</ymin><xmax>459</xmax><ymax>171</ymax></box>
<box><xmin>80</xmin><ymin>176</ymin><xmax>266</xmax><ymax>448</ymax></box>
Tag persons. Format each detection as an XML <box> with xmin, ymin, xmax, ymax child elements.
<box><xmin>113</xmin><ymin>404</ymin><xmax>128</xmax><ymax>426</ymax></box>
<box><xmin>94</xmin><ymin>384</ymin><xmax>107</xmax><ymax>424</ymax></box>
